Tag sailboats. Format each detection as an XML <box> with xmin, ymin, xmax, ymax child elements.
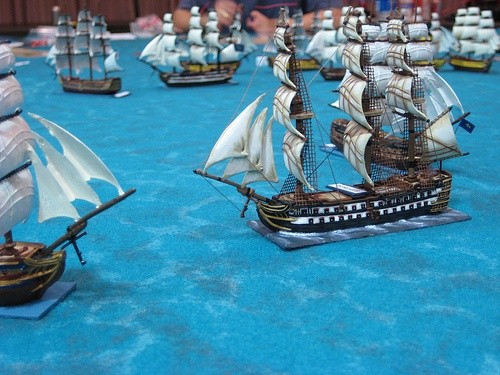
<box><xmin>448</xmin><ymin>6</ymin><xmax>500</xmax><ymax>74</ymax></box>
<box><xmin>0</xmin><ymin>39</ymin><xmax>138</xmax><ymax>308</ymax></box>
<box><xmin>190</xmin><ymin>0</ymin><xmax>470</xmax><ymax>234</ymax></box>
<box><xmin>136</xmin><ymin>6</ymin><xmax>246</xmax><ymax>87</ymax></box>
<box><xmin>262</xmin><ymin>5</ymin><xmax>472</xmax><ymax>172</ymax></box>
<box><xmin>45</xmin><ymin>6</ymin><xmax>124</xmax><ymax>94</ymax></box>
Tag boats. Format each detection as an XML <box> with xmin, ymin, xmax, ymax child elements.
<box><xmin>8</xmin><ymin>25</ymin><xmax>57</xmax><ymax>58</ymax></box>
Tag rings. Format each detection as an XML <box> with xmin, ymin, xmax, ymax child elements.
<box><xmin>219</xmin><ymin>9</ymin><xmax>229</xmax><ymax>19</ymax></box>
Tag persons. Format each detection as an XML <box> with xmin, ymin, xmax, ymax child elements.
<box><xmin>174</xmin><ymin>0</ymin><xmax>345</xmax><ymax>37</ymax></box>
<box><xmin>427</xmin><ymin>0</ymin><xmax>500</xmax><ymax>32</ymax></box>
